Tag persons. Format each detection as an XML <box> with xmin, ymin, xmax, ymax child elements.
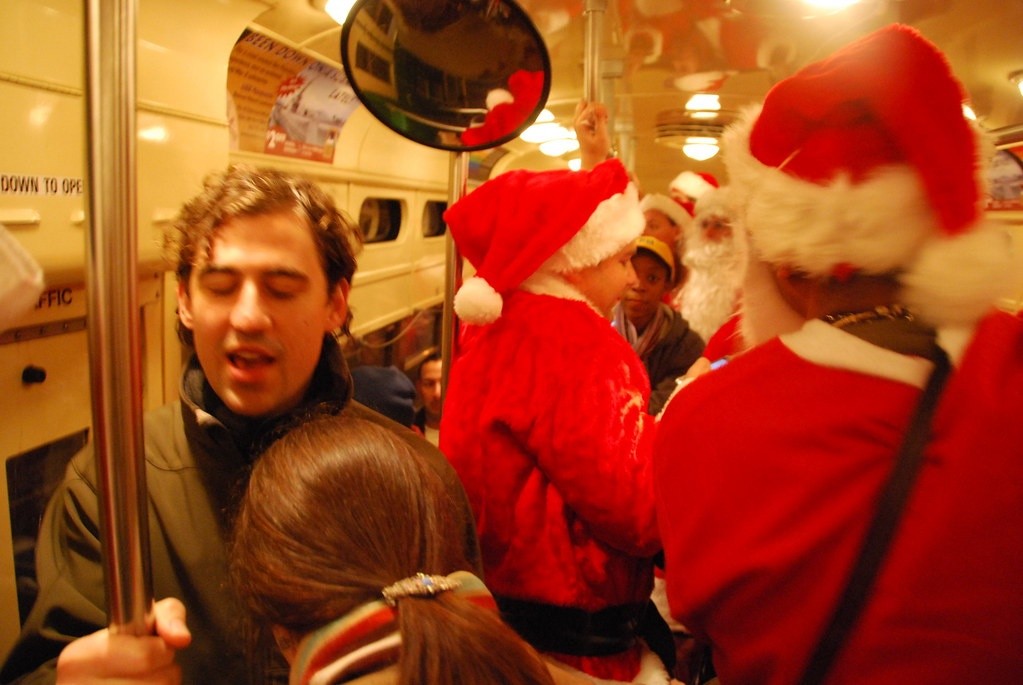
<box><xmin>610</xmin><ymin>171</ymin><xmax>753</xmax><ymax>685</ymax></box>
<box><xmin>439</xmin><ymin>100</ymin><xmax>695</xmax><ymax>685</ymax></box>
<box><xmin>651</xmin><ymin>24</ymin><xmax>1023</xmax><ymax>685</ymax></box>
<box><xmin>452</xmin><ymin>0</ymin><xmax>955</xmax><ymax>93</ymax></box>
<box><xmin>441</xmin><ymin>70</ymin><xmax>546</xmax><ymax>146</ymax></box>
<box><xmin>234</xmin><ymin>418</ymin><xmax>557</xmax><ymax>685</ymax></box>
<box><xmin>415</xmin><ymin>353</ymin><xmax>443</xmax><ymax>449</ymax></box>
<box><xmin>0</xmin><ymin>168</ymin><xmax>474</xmax><ymax>685</ymax></box>
<box><xmin>353</xmin><ymin>364</ymin><xmax>427</xmax><ymax>441</ymax></box>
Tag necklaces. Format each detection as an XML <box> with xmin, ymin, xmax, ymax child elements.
<box><xmin>823</xmin><ymin>304</ymin><xmax>917</xmax><ymax>327</ymax></box>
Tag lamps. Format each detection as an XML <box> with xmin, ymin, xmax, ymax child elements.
<box><xmin>654</xmin><ymin>126</ymin><xmax>725</xmax><ymax>162</ymax></box>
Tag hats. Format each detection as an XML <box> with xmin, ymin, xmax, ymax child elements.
<box><xmin>692</xmin><ymin>185</ymin><xmax>749</xmax><ymax>253</ymax></box>
<box><xmin>637</xmin><ymin>235</ymin><xmax>682</xmax><ymax>285</ymax></box>
<box><xmin>462</xmin><ymin>69</ymin><xmax>546</xmax><ymax>147</ymax></box>
<box><xmin>668</xmin><ymin>170</ymin><xmax>720</xmax><ymax>219</ymax></box>
<box><xmin>639</xmin><ymin>193</ymin><xmax>694</xmax><ymax>234</ymax></box>
<box><xmin>720</xmin><ymin>23</ymin><xmax>1009</xmax><ymax>328</ymax></box>
<box><xmin>443</xmin><ymin>158</ymin><xmax>647</xmax><ymax>324</ymax></box>
